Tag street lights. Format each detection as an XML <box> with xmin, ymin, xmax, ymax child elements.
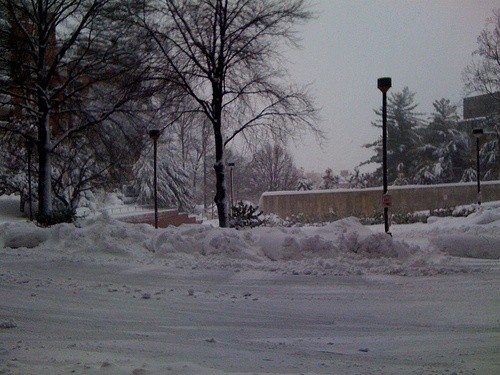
<box><xmin>149</xmin><ymin>128</ymin><xmax>160</xmax><ymax>228</ymax></box>
<box><xmin>227</xmin><ymin>162</ymin><xmax>237</xmax><ymax>215</ymax></box>
<box><xmin>474</xmin><ymin>127</ymin><xmax>483</xmax><ymax>204</ymax></box>
<box><xmin>378</xmin><ymin>76</ymin><xmax>391</xmax><ymax>236</ymax></box>
